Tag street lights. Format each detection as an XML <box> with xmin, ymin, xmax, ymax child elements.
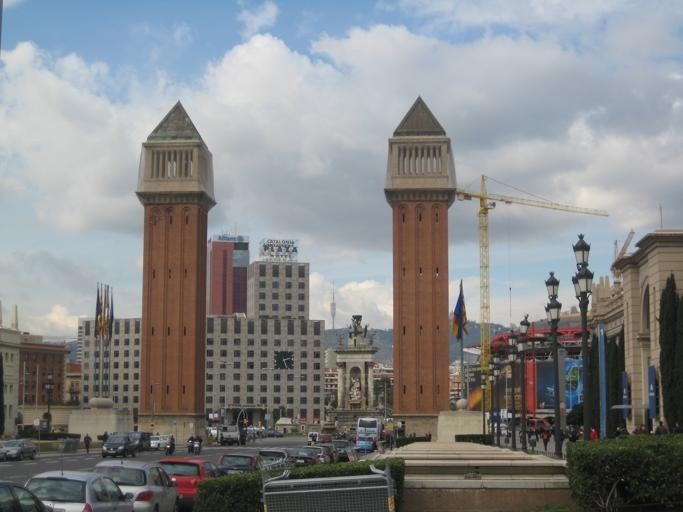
<box><xmin>568</xmin><ymin>232</ymin><xmax>596</xmax><ymax>442</ymax></box>
<box><xmin>43</xmin><ymin>371</ymin><xmax>56</xmax><ymax>432</ymax></box>
<box><xmin>476</xmin><ymin>312</ymin><xmax>533</xmax><ymax>456</ymax></box>
<box><xmin>544</xmin><ymin>270</ymin><xmax>565</xmax><ymax>463</ymax></box>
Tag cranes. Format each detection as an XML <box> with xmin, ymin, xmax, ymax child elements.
<box><xmin>448</xmin><ymin>172</ymin><xmax>611</xmax><ymax>417</ymax></box>
<box><xmin>612</xmin><ymin>227</ymin><xmax>637</xmax><ymax>298</ymax></box>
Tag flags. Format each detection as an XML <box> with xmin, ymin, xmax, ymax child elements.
<box><xmin>451</xmin><ymin>280</ymin><xmax>468</xmax><ymax>342</ymax></box>
<box><xmin>93</xmin><ymin>285</ymin><xmax>113</xmax><ymax>342</ymax></box>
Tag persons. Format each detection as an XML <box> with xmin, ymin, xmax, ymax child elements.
<box><xmin>83</xmin><ymin>433</ymin><xmax>90</xmax><ymax>454</ymax></box>
<box><xmin>193</xmin><ymin>435</ymin><xmax>202</xmax><ymax>444</ymax></box>
<box><xmin>187</xmin><ymin>436</ymin><xmax>193</xmax><ymax>446</ymax></box>
<box><xmin>518</xmin><ymin>421</ymin><xmax>679</xmax><ymax>452</ymax></box>
<box><xmin>167</xmin><ymin>433</ymin><xmax>174</xmax><ymax>448</ymax></box>
